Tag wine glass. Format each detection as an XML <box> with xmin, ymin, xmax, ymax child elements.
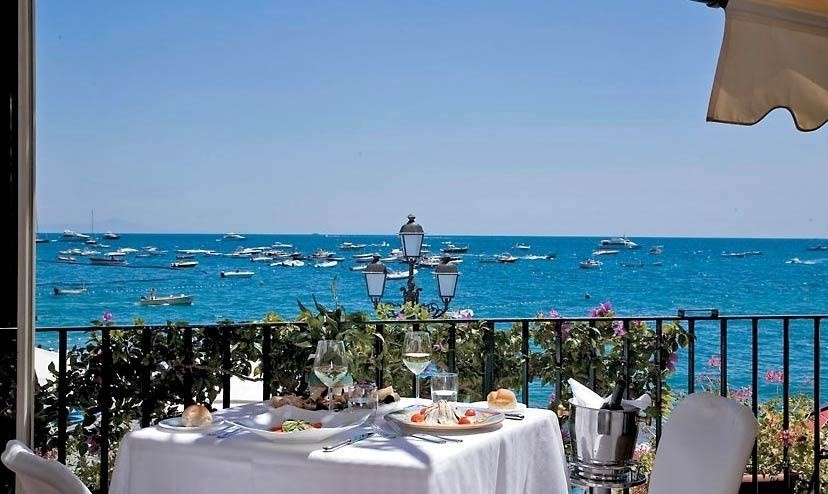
<box><xmin>313</xmin><ymin>341</ymin><xmax>347</xmax><ymax>415</ymax></box>
<box><xmin>401</xmin><ymin>332</ymin><xmax>433</xmax><ymax>405</ymax></box>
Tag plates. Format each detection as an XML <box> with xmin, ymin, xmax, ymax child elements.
<box><xmin>471</xmin><ymin>400</ymin><xmax>527</xmax><ymax>418</ymax></box>
<box><xmin>158</xmin><ymin>416</ymin><xmax>225</xmax><ymax>430</ymax></box>
<box><xmin>226</xmin><ymin>404</ymin><xmax>373</xmax><ymax>442</ymax></box>
<box><xmin>384</xmin><ymin>405</ymin><xmax>506</xmax><ymax>433</ymax></box>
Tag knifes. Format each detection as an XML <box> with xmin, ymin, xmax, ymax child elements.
<box><xmin>322</xmin><ymin>432</ymin><xmax>374</xmax><ymax>450</ymax></box>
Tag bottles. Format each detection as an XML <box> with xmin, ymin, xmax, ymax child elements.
<box><xmin>601</xmin><ymin>379</ymin><xmax>626</xmax><ymax>410</ymax></box>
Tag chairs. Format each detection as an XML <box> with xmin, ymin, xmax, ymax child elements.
<box><xmin>1</xmin><ymin>438</ymin><xmax>92</xmax><ymax>494</ymax></box>
<box><xmin>648</xmin><ymin>391</ymin><xmax>759</xmax><ymax>494</ymax></box>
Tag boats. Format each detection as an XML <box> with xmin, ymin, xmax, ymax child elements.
<box><xmin>579</xmin><ymin>235</ymin><xmax>828</xmax><ymax>268</ymax></box>
<box><xmin>515</xmin><ymin>243</ymin><xmax>530</xmax><ymax>249</ymax></box>
<box><xmin>385</xmin><ymin>268</ymin><xmax>418</xmax><ymax>280</ymax></box>
<box><xmin>141</xmin><ymin>289</ymin><xmax>195</xmax><ymax>305</ymax></box>
<box><xmin>102</xmin><ymin>232</ymin><xmax>120</xmax><ymax>240</ymax></box>
<box><xmin>222</xmin><ymin>233</ymin><xmax>247</xmax><ymax>240</ymax></box>
<box><xmin>35</xmin><ymin>238</ymin><xmax>51</xmax><ymax>243</ymax></box>
<box><xmin>220</xmin><ymin>271</ymin><xmax>256</xmax><ymax>278</ymax></box>
<box><xmin>56</xmin><ymin>228</ymin><xmax>469</xmax><ymax>266</ymax></box>
<box><xmin>497</xmin><ymin>253</ymin><xmax>519</xmax><ymax>263</ymax></box>
<box><xmin>348</xmin><ymin>265</ymin><xmax>368</xmax><ymax>271</ymax></box>
<box><xmin>54</xmin><ymin>287</ymin><xmax>87</xmax><ymax>296</ymax></box>
<box><xmin>743</xmin><ymin>405</ymin><xmax>827</xmax><ymax>494</ymax></box>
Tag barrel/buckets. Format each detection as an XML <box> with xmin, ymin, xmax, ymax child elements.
<box><xmin>567</xmin><ymin>402</ymin><xmax>641</xmax><ymax>483</ymax></box>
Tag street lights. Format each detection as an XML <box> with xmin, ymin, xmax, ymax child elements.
<box><xmin>361</xmin><ymin>212</ymin><xmax>460</xmax><ymax>319</ymax></box>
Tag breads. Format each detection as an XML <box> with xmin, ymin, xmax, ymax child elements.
<box><xmin>487</xmin><ymin>389</ymin><xmax>518</xmax><ymax>410</ymax></box>
<box><xmin>182</xmin><ymin>405</ymin><xmax>213</xmax><ymax>426</ymax></box>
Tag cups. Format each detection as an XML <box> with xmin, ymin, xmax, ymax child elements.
<box><xmin>430</xmin><ymin>374</ymin><xmax>458</xmax><ymax>404</ymax></box>
<box><xmin>350</xmin><ymin>385</ymin><xmax>377</xmax><ymax>416</ymax></box>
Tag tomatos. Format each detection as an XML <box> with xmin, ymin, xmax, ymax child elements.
<box><xmin>465</xmin><ymin>409</ymin><xmax>474</xmax><ymax>416</ymax></box>
<box><xmin>459</xmin><ymin>417</ymin><xmax>470</xmax><ymax>424</ymax></box>
<box><xmin>420</xmin><ymin>408</ymin><xmax>428</xmax><ymax>413</ymax></box>
<box><xmin>410</xmin><ymin>414</ymin><xmax>423</xmax><ymax>421</ymax></box>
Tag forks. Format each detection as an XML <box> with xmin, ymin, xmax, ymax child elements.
<box><xmin>371</xmin><ymin>421</ymin><xmax>465</xmax><ymax>445</ymax></box>
